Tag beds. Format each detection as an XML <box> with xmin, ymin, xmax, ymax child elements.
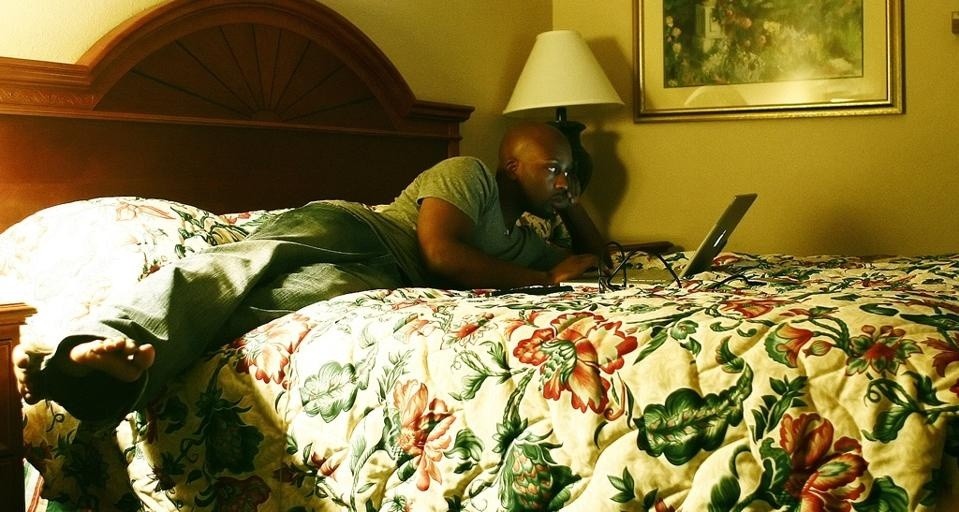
<box><xmin>1</xmin><ymin>0</ymin><xmax>959</xmax><ymax>510</ymax></box>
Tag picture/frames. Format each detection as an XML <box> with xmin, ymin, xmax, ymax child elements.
<box><xmin>631</xmin><ymin>0</ymin><xmax>908</xmax><ymax>126</ymax></box>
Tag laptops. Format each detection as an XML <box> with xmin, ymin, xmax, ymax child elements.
<box><xmin>560</xmin><ymin>193</ymin><xmax>758</xmax><ymax>290</ymax></box>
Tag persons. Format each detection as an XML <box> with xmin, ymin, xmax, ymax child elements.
<box><xmin>9</xmin><ymin>121</ymin><xmax>613</xmax><ymax>429</ymax></box>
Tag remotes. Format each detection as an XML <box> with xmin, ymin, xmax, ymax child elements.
<box><xmin>492</xmin><ymin>284</ymin><xmax>573</xmax><ymax>296</ymax></box>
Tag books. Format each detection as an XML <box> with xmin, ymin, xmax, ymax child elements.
<box><xmin>489</xmin><ymin>285</ymin><xmax>574</xmax><ymax>299</ymax></box>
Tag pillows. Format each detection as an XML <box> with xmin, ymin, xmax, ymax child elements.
<box><xmin>2</xmin><ymin>194</ymin><xmax>247</xmax><ymax>325</ymax></box>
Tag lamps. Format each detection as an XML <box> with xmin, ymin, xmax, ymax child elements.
<box><xmin>503</xmin><ymin>26</ymin><xmax>628</xmax><ymax>196</ymax></box>
